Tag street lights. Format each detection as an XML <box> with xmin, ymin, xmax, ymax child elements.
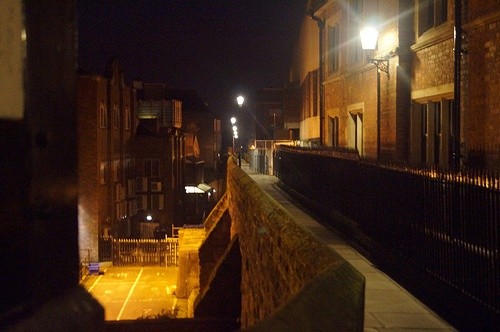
<box><xmin>231</xmin><ymin>96</ymin><xmax>244</xmax><ymax>168</ymax></box>
